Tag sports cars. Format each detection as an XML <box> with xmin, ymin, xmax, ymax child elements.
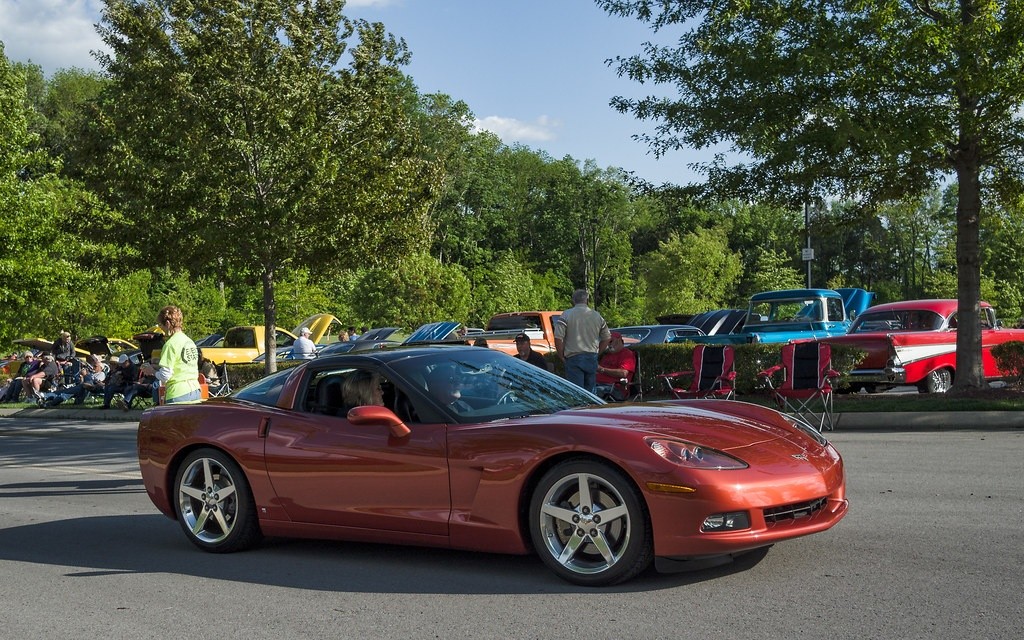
<box><xmin>137</xmin><ymin>342</ymin><xmax>851</xmax><ymax>586</ymax></box>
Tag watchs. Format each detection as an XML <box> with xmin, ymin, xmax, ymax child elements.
<box><xmin>601</xmin><ymin>368</ymin><xmax>605</xmax><ymax>372</ymax></box>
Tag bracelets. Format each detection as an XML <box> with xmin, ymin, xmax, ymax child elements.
<box><xmin>152</xmin><ymin>371</ymin><xmax>155</xmax><ymax>376</ymax></box>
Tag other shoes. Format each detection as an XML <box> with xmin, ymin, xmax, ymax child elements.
<box><xmin>99</xmin><ymin>404</ymin><xmax>110</xmax><ymax>409</ymax></box>
<box><xmin>114</xmin><ymin>399</ymin><xmax>129</xmax><ymax>413</ymax></box>
<box><xmin>24</xmin><ymin>397</ymin><xmax>37</xmax><ymax>403</ymax></box>
<box><xmin>31</xmin><ymin>387</ymin><xmax>46</xmax><ymax>408</ymax></box>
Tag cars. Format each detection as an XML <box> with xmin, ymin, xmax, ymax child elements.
<box><xmin>786</xmin><ymin>299</ymin><xmax>1024</xmax><ymax>394</ymax></box>
<box><xmin>664</xmin><ymin>287</ymin><xmax>878</xmax><ymax>344</ymax></box>
<box><xmin>0</xmin><ymin>312</ymin><xmax>642</xmax><ymax>387</ymax></box>
<box><xmin>606</xmin><ymin>308</ymin><xmax>749</xmax><ymax>348</ymax></box>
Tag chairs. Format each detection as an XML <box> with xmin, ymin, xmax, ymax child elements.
<box><xmin>309</xmin><ymin>374</ymin><xmax>348</xmax><ymax>419</ymax></box>
<box><xmin>21</xmin><ymin>352</ymin><xmax>158</xmax><ymax>411</ymax></box>
<box><xmin>657</xmin><ymin>345</ymin><xmax>737</xmax><ymax>401</ymax></box>
<box><xmin>394</xmin><ymin>368</ymin><xmax>425</xmax><ymax>424</ymax></box>
<box><xmin>756</xmin><ymin>341</ymin><xmax>842</xmax><ymax>434</ymax></box>
<box><xmin>604</xmin><ymin>350</ymin><xmax>644</xmax><ymax>403</ymax></box>
<box><xmin>208</xmin><ymin>360</ymin><xmax>234</xmax><ymax>397</ymax></box>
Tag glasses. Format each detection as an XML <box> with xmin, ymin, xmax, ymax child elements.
<box><xmin>67</xmin><ymin>335</ymin><xmax>72</xmax><ymax>338</ymax></box>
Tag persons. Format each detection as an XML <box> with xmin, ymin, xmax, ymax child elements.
<box><xmin>554</xmin><ymin>290</ymin><xmax>611</xmax><ymax>395</ymax></box>
<box><xmin>428</xmin><ymin>365</ymin><xmax>475</xmax><ymax>414</ymax></box>
<box><xmin>461</xmin><ymin>326</ymin><xmax>548</xmax><ymax>371</ymax></box>
<box><xmin>141</xmin><ymin>307</ymin><xmax>202</xmax><ymax>404</ymax></box>
<box><xmin>0</xmin><ymin>331</ymin><xmax>222</xmax><ymax>411</ymax></box>
<box><xmin>293</xmin><ymin>327</ymin><xmax>318</xmax><ymax>360</ymax></box>
<box><xmin>340</xmin><ymin>370</ymin><xmax>385</xmax><ymax>418</ymax></box>
<box><xmin>595</xmin><ymin>332</ymin><xmax>636</xmax><ymax>403</ymax></box>
<box><xmin>338</xmin><ymin>327</ymin><xmax>368</xmax><ymax>341</ymax></box>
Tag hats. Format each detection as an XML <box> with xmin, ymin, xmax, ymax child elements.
<box><xmin>611</xmin><ymin>331</ymin><xmax>622</xmax><ymax>339</ymax></box>
<box><xmin>300</xmin><ymin>327</ymin><xmax>313</xmax><ymax>334</ymax></box>
<box><xmin>513</xmin><ymin>333</ymin><xmax>530</xmax><ymax>342</ymax></box>
<box><xmin>39</xmin><ymin>351</ymin><xmax>50</xmax><ymax>357</ymax></box>
<box><xmin>119</xmin><ymin>354</ymin><xmax>128</xmax><ymax>363</ymax></box>
<box><xmin>109</xmin><ymin>356</ymin><xmax>119</xmax><ymax>364</ymax></box>
